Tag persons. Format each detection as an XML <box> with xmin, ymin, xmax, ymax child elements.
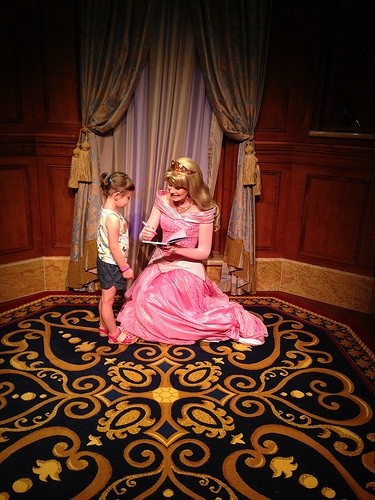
<box><xmin>97</xmin><ymin>172</ymin><xmax>138</xmax><ymax>344</ymax></box>
<box><xmin>116</xmin><ymin>158</ymin><xmax>269</xmax><ymax>346</ymax></box>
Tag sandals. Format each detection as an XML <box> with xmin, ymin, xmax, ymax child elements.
<box><xmin>98</xmin><ymin>327</ymin><xmax>124</xmax><ymax>337</ymax></box>
<box><xmin>108</xmin><ymin>329</ymin><xmax>138</xmax><ymax>344</ymax></box>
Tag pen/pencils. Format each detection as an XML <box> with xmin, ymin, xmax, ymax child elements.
<box><xmin>142</xmin><ymin>221</ymin><xmax>158</xmax><ymax>235</ymax></box>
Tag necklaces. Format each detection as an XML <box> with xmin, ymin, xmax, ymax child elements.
<box><xmin>171</xmin><ymin>199</ymin><xmax>194</xmax><ymax>214</ymax></box>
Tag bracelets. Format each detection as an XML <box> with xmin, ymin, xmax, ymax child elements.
<box><xmin>173</xmin><ymin>247</ymin><xmax>176</xmax><ymax>254</ymax></box>
<box><xmin>120</xmin><ymin>264</ymin><xmax>130</xmax><ymax>272</ymax></box>
